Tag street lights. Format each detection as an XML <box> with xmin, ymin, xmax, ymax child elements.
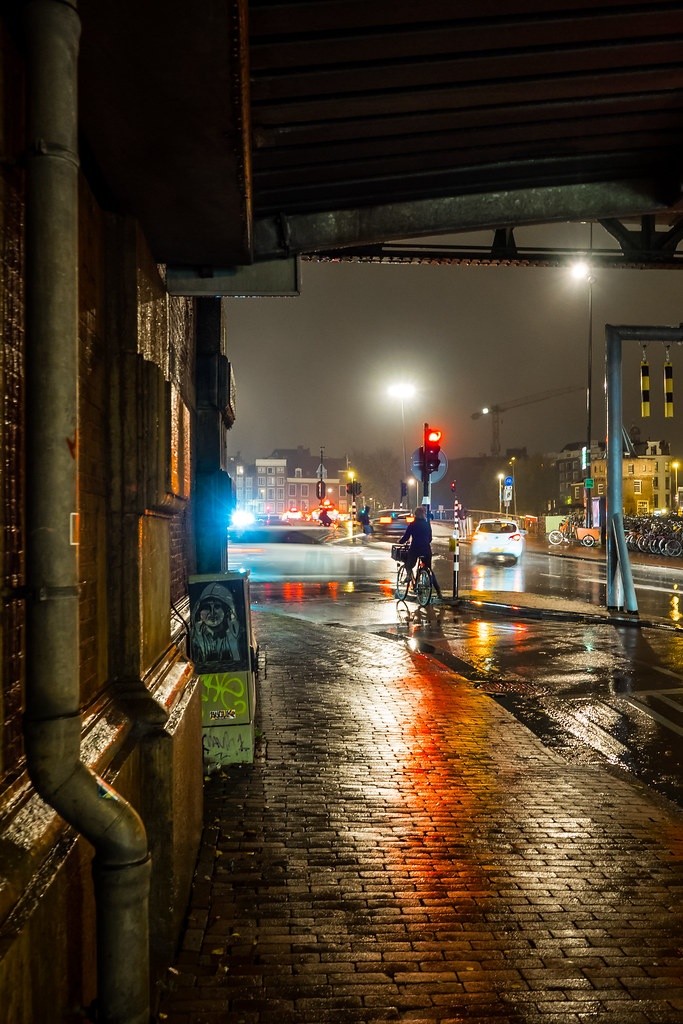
<box><xmin>673</xmin><ymin>461</ymin><xmax>680</xmax><ymax>493</ymax></box>
<box><xmin>349</xmin><ymin>471</ymin><xmax>354</xmax><ymax>520</ymax></box>
<box><xmin>498</xmin><ymin>473</ymin><xmax>504</xmax><ymax>513</ymax></box>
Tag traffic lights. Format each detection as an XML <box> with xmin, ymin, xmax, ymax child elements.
<box><xmin>450</xmin><ymin>482</ymin><xmax>456</xmax><ymax>492</ymax></box>
<box><xmin>402</xmin><ymin>482</ymin><xmax>407</xmax><ymax>496</ymax></box>
<box><xmin>426</xmin><ymin>429</ymin><xmax>442</xmax><ymax>473</ymax></box>
<box><xmin>409</xmin><ymin>478</ymin><xmax>419</xmax><ymax>507</ymax></box>
<box><xmin>357</xmin><ymin>482</ymin><xmax>362</xmax><ymax>495</ymax></box>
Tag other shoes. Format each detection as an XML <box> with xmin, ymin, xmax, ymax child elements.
<box><xmin>404</xmin><ymin>576</ymin><xmax>412</xmax><ymax>583</ymax></box>
<box><xmin>459</xmin><ymin>536</ymin><xmax>467</xmax><ymax>539</ymax></box>
<box><xmin>434</xmin><ymin>594</ymin><xmax>442</xmax><ymax>599</ymax></box>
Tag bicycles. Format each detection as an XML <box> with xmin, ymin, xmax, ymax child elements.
<box><xmin>547</xmin><ymin>507</ymin><xmax>683</xmax><ymax>557</ymax></box>
<box><xmin>391</xmin><ymin>541</ymin><xmax>433</xmax><ymax>607</ymax></box>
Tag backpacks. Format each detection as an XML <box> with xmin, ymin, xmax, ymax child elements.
<box><xmin>356</xmin><ymin>510</ymin><xmax>364</xmax><ymax>522</ymax></box>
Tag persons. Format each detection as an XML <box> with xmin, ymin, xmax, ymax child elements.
<box><xmin>398</xmin><ymin>507</ymin><xmax>442</xmax><ymax>600</ymax></box>
<box><xmin>457</xmin><ymin>504</ymin><xmax>467</xmax><ymax>539</ymax></box>
<box><xmin>361</xmin><ymin>506</ymin><xmax>372</xmax><ymax>544</ymax></box>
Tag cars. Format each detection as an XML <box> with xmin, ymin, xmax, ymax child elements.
<box><xmin>471</xmin><ymin>517</ymin><xmax>526</xmax><ymax>563</ymax></box>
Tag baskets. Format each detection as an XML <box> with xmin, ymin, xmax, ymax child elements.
<box><xmin>391</xmin><ymin>545</ymin><xmax>410</xmax><ymax>561</ymax></box>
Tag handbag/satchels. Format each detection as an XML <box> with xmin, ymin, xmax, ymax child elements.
<box><xmin>364</xmin><ymin>524</ymin><xmax>373</xmax><ymax>534</ymax></box>
